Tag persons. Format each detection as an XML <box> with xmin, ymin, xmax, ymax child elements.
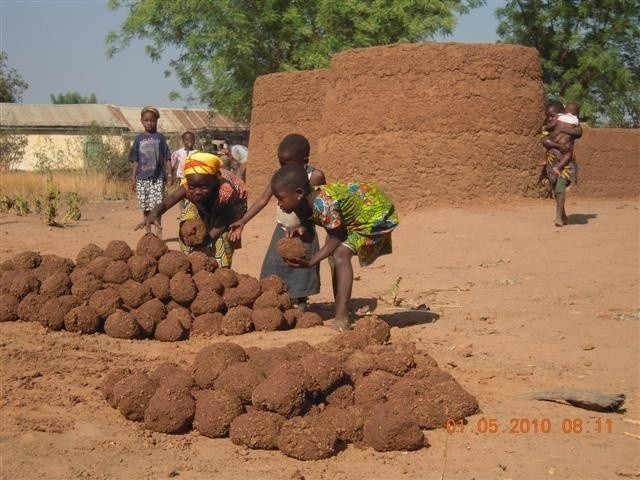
<box><xmin>542</xmin><ymin>103</ymin><xmax>582</xmax><ymax>186</ymax></box>
<box><xmin>127</xmin><ymin>106</ymin><xmax>173</xmax><ymax>242</ymax></box>
<box><xmin>135</xmin><ymin>152</ymin><xmax>246</xmax><ymax>274</ymax></box>
<box><xmin>167</xmin><ymin>131</ymin><xmax>196</xmax><ymax>210</ymax></box>
<box><xmin>540</xmin><ymin>101</ymin><xmax>583</xmax><ymax>227</ymax></box>
<box><xmin>270</xmin><ymin>163</ymin><xmax>399</xmax><ymax>329</ymax></box>
<box><xmin>228</xmin><ymin>133</ymin><xmax>327</xmax><ymax>310</ymax></box>
<box><xmin>213</xmin><ymin>141</ymin><xmax>250</xmax><ymax>182</ymax></box>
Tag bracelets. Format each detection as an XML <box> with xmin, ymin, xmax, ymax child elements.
<box><xmin>167</xmin><ymin>175</ymin><xmax>173</xmax><ymax>177</ymax></box>
